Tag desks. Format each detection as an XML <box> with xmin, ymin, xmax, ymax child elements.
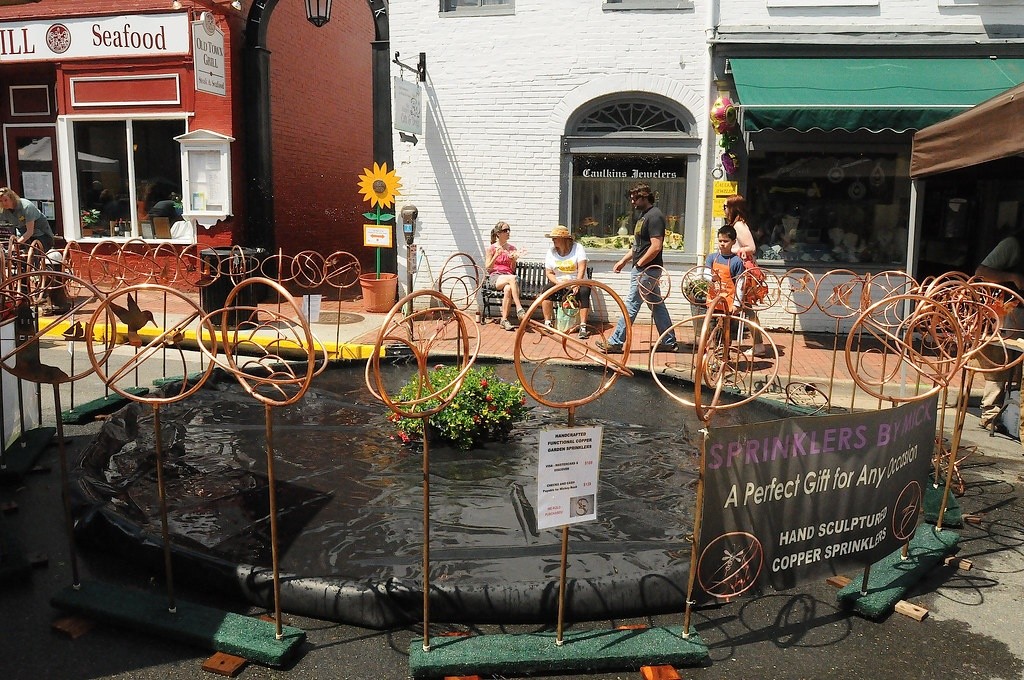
<box><xmin>935</xmin><ymin>332</ymin><xmax>1024</xmax><ymax>436</ymax></box>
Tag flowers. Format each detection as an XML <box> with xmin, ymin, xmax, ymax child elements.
<box><xmin>356</xmin><ymin>160</ymin><xmax>400</xmax><ymax>280</ymax></box>
<box><xmin>80</xmin><ymin>208</ymin><xmax>101</xmax><ymax>229</ymax></box>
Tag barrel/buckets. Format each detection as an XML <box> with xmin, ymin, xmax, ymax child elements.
<box><xmin>301</xmin><ymin>295</ymin><xmax>322</xmax><ymax>322</ymax></box>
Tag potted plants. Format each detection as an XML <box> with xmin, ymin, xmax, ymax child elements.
<box><xmin>685</xmin><ymin>280</ymin><xmax>731</xmax><ymax>340</ymax></box>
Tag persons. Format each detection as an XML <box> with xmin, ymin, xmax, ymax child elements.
<box><xmin>974</xmin><ymin>235</ymin><xmax>1024</xmax><ymax>432</ymax></box>
<box><xmin>704</xmin><ymin>225</ymin><xmax>745</xmax><ymax>371</ymax></box>
<box><xmin>595</xmin><ymin>183</ymin><xmax>679</xmax><ymax>353</ymax></box>
<box><xmin>146</xmin><ymin>200</ymin><xmax>182</xmax><ymax>231</ymax></box>
<box><xmin>0</xmin><ymin>187</ymin><xmax>54</xmax><ymax>295</ymax></box>
<box><xmin>100</xmin><ymin>190</ymin><xmax>116</xmax><ymax>220</ymax></box>
<box><xmin>541</xmin><ymin>225</ymin><xmax>591</xmax><ymax>338</ymax></box>
<box><xmin>711</xmin><ymin>194</ymin><xmax>766</xmax><ymax>360</ymax></box>
<box><xmin>486</xmin><ymin>221</ymin><xmax>525</xmax><ymax>331</ymax></box>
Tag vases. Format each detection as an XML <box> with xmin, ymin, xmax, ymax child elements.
<box><xmin>83</xmin><ymin>229</ymin><xmax>94</xmax><ymax>235</ymax></box>
<box><xmin>358</xmin><ymin>272</ymin><xmax>398</xmax><ymax>313</ymax></box>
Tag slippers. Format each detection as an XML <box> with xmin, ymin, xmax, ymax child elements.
<box><xmin>743</xmin><ymin>348</ymin><xmax>766</xmax><ymax>355</ymax></box>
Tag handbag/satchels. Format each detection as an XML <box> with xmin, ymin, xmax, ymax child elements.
<box><xmin>557</xmin><ymin>294</ymin><xmax>580</xmax><ymax>332</ymax></box>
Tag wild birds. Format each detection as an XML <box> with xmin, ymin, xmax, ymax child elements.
<box><xmin>107</xmin><ymin>292</ymin><xmax>159</xmax><ymax>331</ymax></box>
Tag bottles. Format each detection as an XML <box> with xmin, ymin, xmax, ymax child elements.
<box><xmin>114</xmin><ymin>218</ymin><xmax>130</xmax><ymax>237</ymax></box>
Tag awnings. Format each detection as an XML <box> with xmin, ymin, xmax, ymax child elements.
<box><xmin>722</xmin><ymin>55</ymin><xmax>1024</xmax><ymax>136</ymax></box>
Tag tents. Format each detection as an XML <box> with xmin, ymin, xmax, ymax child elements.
<box><xmin>902</xmin><ymin>82</ymin><xmax>1024</xmax><ymax>328</ymax></box>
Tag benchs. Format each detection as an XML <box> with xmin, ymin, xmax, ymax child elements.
<box><xmin>481</xmin><ymin>262</ymin><xmax>592</xmax><ymax>325</ymax></box>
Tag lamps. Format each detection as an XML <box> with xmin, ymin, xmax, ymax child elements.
<box><xmin>304</xmin><ymin>0</ymin><xmax>332</xmax><ymax>26</ymax></box>
<box><xmin>231</xmin><ymin>0</ymin><xmax>241</xmax><ymax>10</ymax></box>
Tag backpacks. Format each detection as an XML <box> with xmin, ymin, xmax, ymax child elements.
<box><xmin>737</xmin><ymin>249</ymin><xmax>768</xmax><ymax>306</ymax></box>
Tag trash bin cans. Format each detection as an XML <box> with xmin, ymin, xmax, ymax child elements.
<box><xmin>199</xmin><ymin>247</ymin><xmax>256</xmax><ymax>330</ymax></box>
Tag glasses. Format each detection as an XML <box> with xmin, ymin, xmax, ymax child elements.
<box><xmin>724</xmin><ymin>204</ymin><xmax>727</xmax><ymax>210</ymax></box>
<box><xmin>501</xmin><ymin>228</ymin><xmax>510</xmax><ymax>232</ymax></box>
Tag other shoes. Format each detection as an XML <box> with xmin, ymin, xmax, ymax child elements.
<box><xmin>578</xmin><ymin>329</ymin><xmax>589</xmax><ymax>338</ymax></box>
<box><xmin>517</xmin><ymin>308</ymin><xmax>526</xmax><ymax>321</ymax></box>
<box><xmin>499</xmin><ymin>319</ymin><xmax>515</xmax><ymax>331</ymax></box>
<box><xmin>979</xmin><ymin>417</ymin><xmax>1004</xmax><ymax>433</ymax></box>
<box><xmin>539</xmin><ymin>323</ymin><xmax>555</xmax><ymax>336</ymax></box>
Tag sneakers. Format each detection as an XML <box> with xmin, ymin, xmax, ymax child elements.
<box><xmin>595</xmin><ymin>339</ymin><xmax>623</xmax><ymax>353</ymax></box>
<box><xmin>651</xmin><ymin>342</ymin><xmax>680</xmax><ymax>352</ymax></box>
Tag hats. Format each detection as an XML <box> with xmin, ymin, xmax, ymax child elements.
<box><xmin>545</xmin><ymin>225</ymin><xmax>573</xmax><ymax>238</ymax></box>
